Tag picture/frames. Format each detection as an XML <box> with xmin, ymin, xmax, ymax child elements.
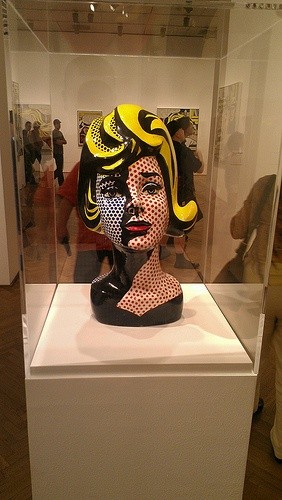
<box><xmin>76</xmin><ymin>110</ymin><xmax>102</xmax><ymax>146</ymax></box>
<box><xmin>12</xmin><ymin>103</ymin><xmax>53</xmax><ymax>156</ymax></box>
<box><xmin>157</xmin><ymin>107</ymin><xmax>199</xmax><ymax>157</ymax></box>
<box><xmin>213</xmin><ymin>81</ymin><xmax>242</xmax><ymax>167</ymax></box>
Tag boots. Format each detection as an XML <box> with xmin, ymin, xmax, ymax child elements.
<box><xmin>174</xmin><ymin>254</ymin><xmax>200</xmax><ymax>270</ymax></box>
<box><xmin>159</xmin><ymin>246</ymin><xmax>171</xmax><ymax>260</ymax></box>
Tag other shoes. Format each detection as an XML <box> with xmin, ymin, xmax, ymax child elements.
<box><xmin>270</xmin><ymin>442</ymin><xmax>282</xmax><ymax>465</ymax></box>
<box><xmin>254</xmin><ymin>399</ymin><xmax>264</xmax><ymax>419</ymax></box>
<box><xmin>167</xmin><ymin>235</ymin><xmax>189</xmax><ymax>244</ymax></box>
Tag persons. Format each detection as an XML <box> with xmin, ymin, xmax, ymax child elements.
<box><xmin>224</xmin><ymin>171</ymin><xmax>282</xmax><ymax>464</ymax></box>
<box><xmin>22</xmin><ymin>121</ymin><xmax>39</xmax><ymax>185</ymax></box>
<box><xmin>51</xmin><ymin>118</ymin><xmax>67</xmax><ymax>182</ymax></box>
<box><xmin>176</xmin><ymin>116</ymin><xmax>195</xmax><ymax>144</ymax></box>
<box><xmin>50</xmin><ymin>160</ymin><xmax>115</xmax><ymax>284</ymax></box>
<box><xmin>30</xmin><ymin>121</ymin><xmax>50</xmax><ymax>176</ymax></box>
<box><xmin>74</xmin><ymin>105</ymin><xmax>202</xmax><ymax>326</ymax></box>
<box><xmin>162</xmin><ymin>119</ymin><xmax>205</xmax><ymax>271</ymax></box>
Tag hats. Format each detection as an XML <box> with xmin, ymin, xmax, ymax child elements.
<box><xmin>53</xmin><ymin>119</ymin><xmax>61</xmax><ymax>125</ymax></box>
<box><xmin>166</xmin><ymin>121</ymin><xmax>182</xmax><ymax>138</ymax></box>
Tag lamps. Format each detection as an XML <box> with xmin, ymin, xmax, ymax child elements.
<box><xmin>90</xmin><ymin>4</ymin><xmax>98</xmax><ymax>12</ymax></box>
<box><xmin>88</xmin><ymin>13</ymin><xmax>93</xmax><ymax>23</ymax></box>
<box><xmin>184</xmin><ymin>16</ymin><xmax>190</xmax><ymax>27</ymax></box>
<box><xmin>72</xmin><ymin>13</ymin><xmax>79</xmax><ymax>22</ymax></box>
<box><xmin>118</xmin><ymin>26</ymin><xmax>122</xmax><ymax>36</ymax></box>
<box><xmin>160</xmin><ymin>28</ymin><xmax>166</xmax><ymax>37</ymax></box>
<box><xmin>110</xmin><ymin>4</ymin><xmax>119</xmax><ymax>11</ymax></box>
<box><xmin>183</xmin><ymin>7</ymin><xmax>193</xmax><ymax>13</ymax></box>
